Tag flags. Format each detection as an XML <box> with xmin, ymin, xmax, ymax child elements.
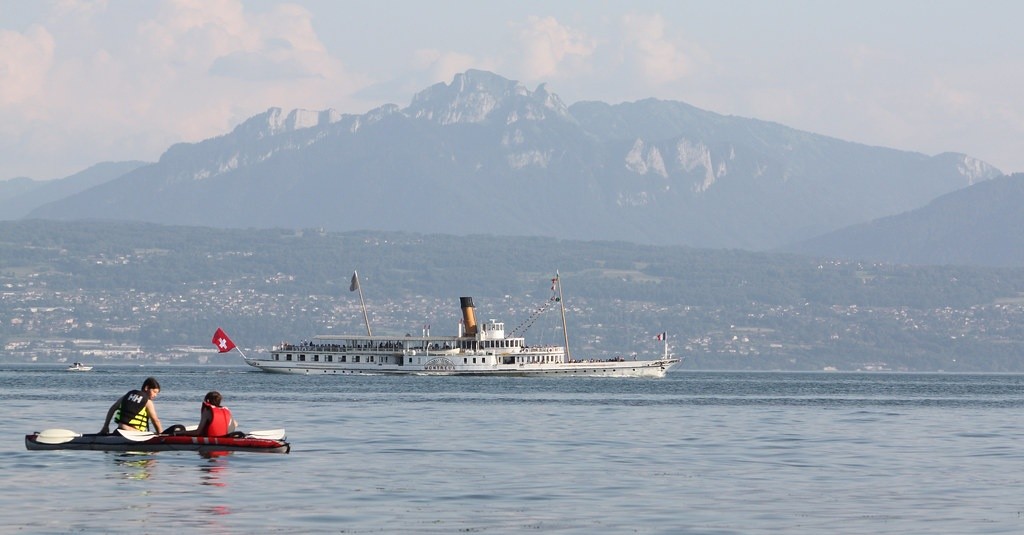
<box><xmin>212</xmin><ymin>328</ymin><xmax>236</xmax><ymax>353</ymax></box>
<box><xmin>350</xmin><ymin>273</ymin><xmax>358</xmax><ymax>291</ymax></box>
<box><xmin>658</xmin><ymin>333</ymin><xmax>665</xmax><ymax>340</ymax></box>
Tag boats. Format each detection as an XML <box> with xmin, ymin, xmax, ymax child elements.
<box><xmin>66</xmin><ymin>361</ymin><xmax>93</xmax><ymax>372</ymax></box>
<box><xmin>25</xmin><ymin>431</ymin><xmax>291</xmax><ymax>455</ymax></box>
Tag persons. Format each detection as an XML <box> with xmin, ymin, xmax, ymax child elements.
<box><xmin>521</xmin><ymin>344</ymin><xmax>557</xmax><ymax>352</ymax></box>
<box><xmin>281</xmin><ymin>339</ymin><xmax>402</xmax><ymax>350</ymax></box>
<box><xmin>97</xmin><ymin>377</ymin><xmax>163</xmax><ymax>435</ymax></box>
<box><xmin>443</xmin><ymin>343</ymin><xmax>446</xmax><ymax>349</ymax></box>
<box><xmin>429</xmin><ymin>344</ymin><xmax>439</xmax><ymax>350</ymax></box>
<box><xmin>183</xmin><ymin>391</ymin><xmax>238</xmax><ymax>436</ymax></box>
<box><xmin>569</xmin><ymin>356</ymin><xmax>625</xmax><ymax>363</ymax></box>
<box><xmin>520</xmin><ymin>360</ymin><xmax>562</xmax><ymax>365</ymax></box>
<box><xmin>634</xmin><ymin>355</ymin><xmax>637</xmax><ymax>361</ymax></box>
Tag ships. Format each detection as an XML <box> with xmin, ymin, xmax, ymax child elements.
<box><xmin>244</xmin><ymin>295</ymin><xmax>680</xmax><ymax>378</ymax></box>
